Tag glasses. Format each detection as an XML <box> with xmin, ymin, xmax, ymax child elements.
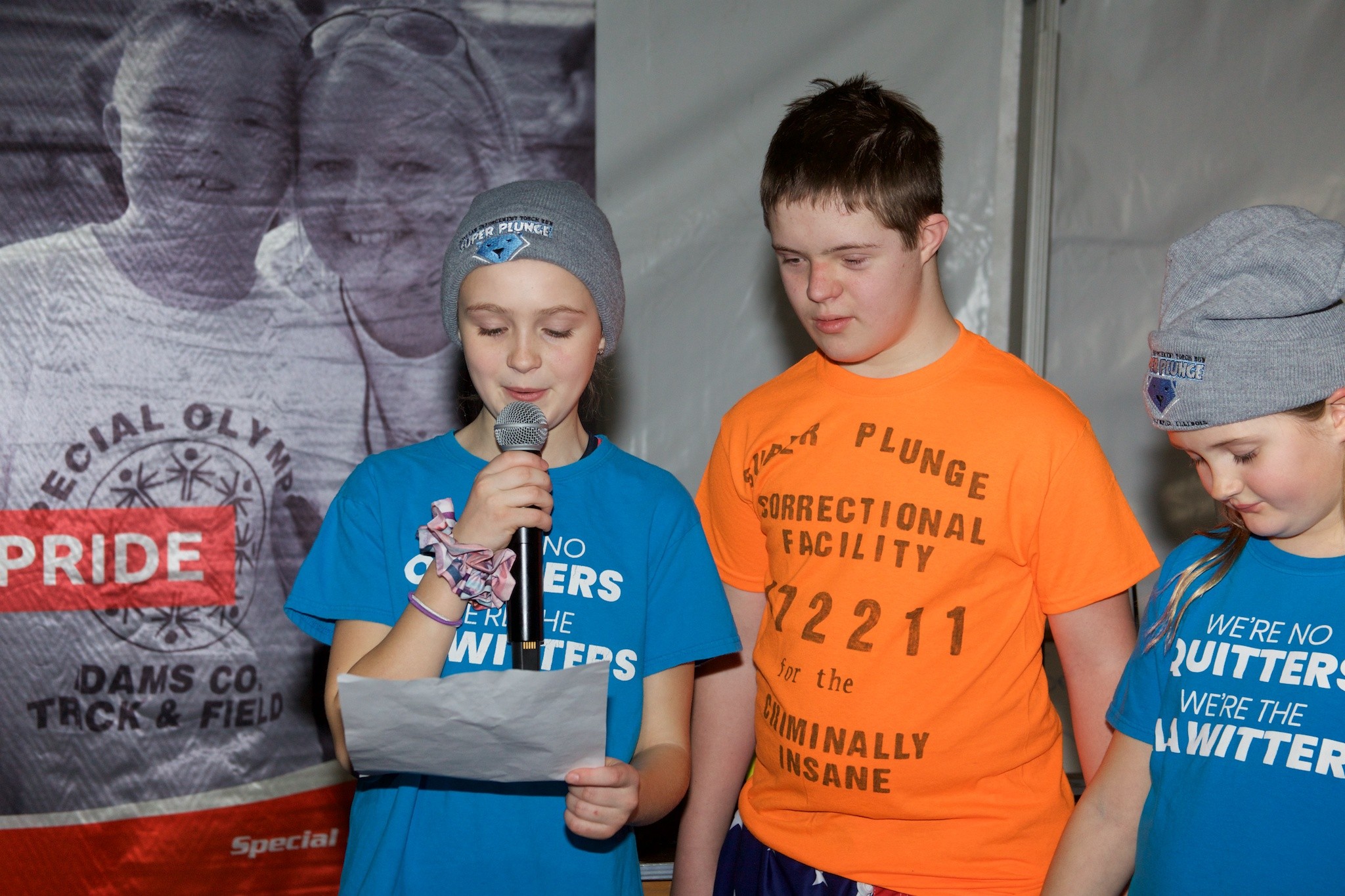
<box><xmin>304</xmin><ymin>6</ymin><xmax>488</xmax><ymax>97</ymax></box>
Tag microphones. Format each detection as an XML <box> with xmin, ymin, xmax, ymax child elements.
<box><xmin>493</xmin><ymin>399</ymin><xmax>549</xmax><ymax>650</ymax></box>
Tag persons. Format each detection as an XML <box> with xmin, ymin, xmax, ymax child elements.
<box><xmin>1034</xmin><ymin>202</ymin><xmax>1345</xmax><ymax>895</ymax></box>
<box><xmin>253</xmin><ymin>8</ymin><xmax>529</xmax><ymax>448</ymax></box>
<box><xmin>282</xmin><ymin>181</ymin><xmax>743</xmax><ymax>896</ymax></box>
<box><xmin>668</xmin><ymin>73</ymin><xmax>1162</xmax><ymax>896</ymax></box>
<box><xmin>1</xmin><ymin>0</ymin><xmax>375</xmax><ymax>820</ymax></box>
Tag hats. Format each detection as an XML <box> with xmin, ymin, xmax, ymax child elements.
<box><xmin>1137</xmin><ymin>211</ymin><xmax>1344</xmax><ymax>429</ymax></box>
<box><xmin>435</xmin><ymin>181</ymin><xmax>627</xmax><ymax>371</ymax></box>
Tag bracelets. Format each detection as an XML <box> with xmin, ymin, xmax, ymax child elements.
<box><xmin>408</xmin><ymin>593</ymin><xmax>463</xmax><ymax>629</ymax></box>
<box><xmin>414</xmin><ymin>498</ymin><xmax>516</xmax><ymax>611</ymax></box>
